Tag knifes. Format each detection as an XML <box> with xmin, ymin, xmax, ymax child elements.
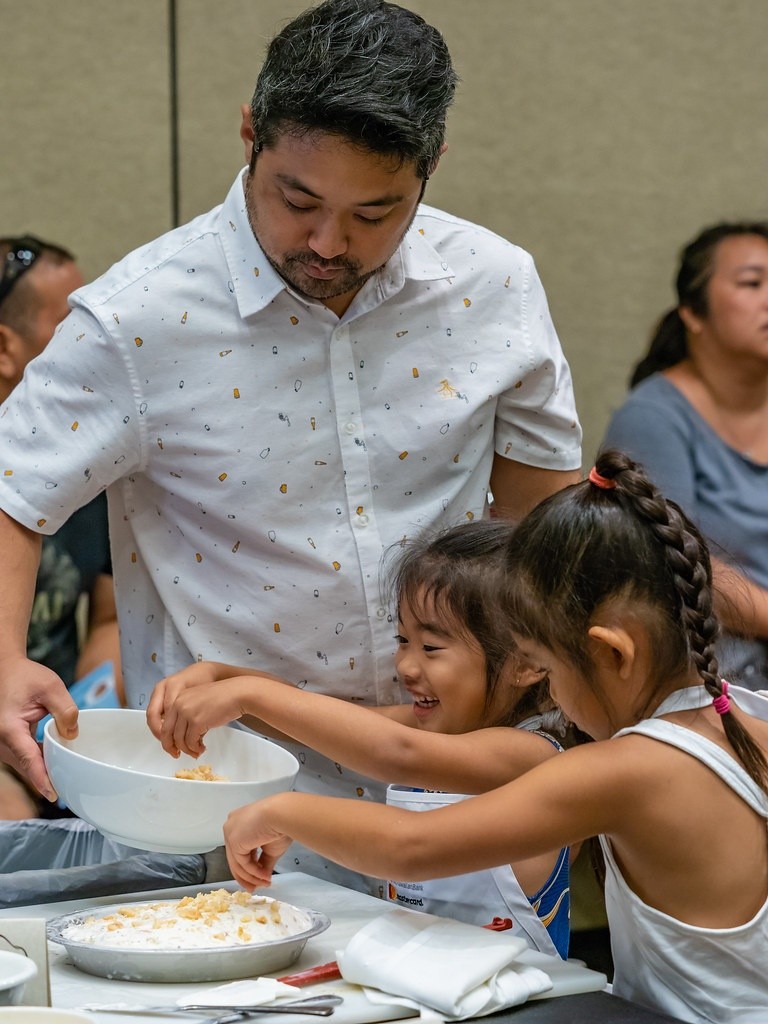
<box><xmin>78</xmin><ymin>1001</ymin><xmax>333</xmax><ymax>1017</ymax></box>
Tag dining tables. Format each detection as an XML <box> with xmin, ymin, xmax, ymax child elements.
<box><xmin>0</xmin><ymin>870</ymin><xmax>684</xmax><ymax>1024</ymax></box>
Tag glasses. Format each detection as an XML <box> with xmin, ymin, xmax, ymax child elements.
<box><xmin>0</xmin><ymin>237</ymin><xmax>44</xmax><ymax>303</ymax></box>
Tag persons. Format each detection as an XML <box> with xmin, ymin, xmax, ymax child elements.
<box><xmin>1</xmin><ymin>234</ymin><xmax>125</xmax><ymax>818</ymax></box>
<box><xmin>1</xmin><ymin>4</ymin><xmax>583</xmax><ymax>894</ymax></box>
<box><xmin>146</xmin><ymin>521</ymin><xmax>573</xmax><ymax>962</ymax></box>
<box><xmin>224</xmin><ymin>451</ymin><xmax>768</xmax><ymax>1024</ymax></box>
<box><xmin>595</xmin><ymin>221</ymin><xmax>767</xmax><ymax>694</ymax></box>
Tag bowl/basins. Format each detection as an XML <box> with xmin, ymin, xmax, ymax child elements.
<box><xmin>45</xmin><ymin>898</ymin><xmax>330</xmax><ymax>983</ymax></box>
<box><xmin>0</xmin><ymin>950</ymin><xmax>37</xmax><ymax>1006</ymax></box>
<box><xmin>42</xmin><ymin>709</ymin><xmax>300</xmax><ymax>856</ymax></box>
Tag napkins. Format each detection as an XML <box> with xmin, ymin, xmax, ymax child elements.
<box><xmin>176</xmin><ymin>977</ymin><xmax>310</xmax><ymax>1015</ymax></box>
<box><xmin>335</xmin><ymin>910</ymin><xmax>554</xmax><ymax>1022</ymax></box>
